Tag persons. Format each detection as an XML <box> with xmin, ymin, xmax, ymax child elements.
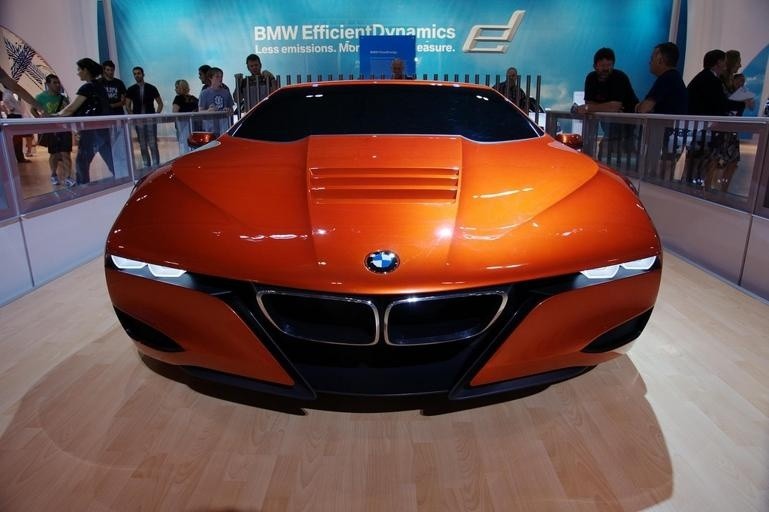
<box><xmin>571</xmin><ymin>48</ymin><xmax>639</xmax><ymax>160</ymax></box>
<box><xmin>0</xmin><ymin>53</ymin><xmax>279</xmax><ymax>189</ymax></box>
<box><xmin>635</xmin><ymin>42</ymin><xmax>687</xmax><ymax>181</ymax></box>
<box><xmin>392</xmin><ymin>58</ymin><xmax>411</xmax><ymax>80</ymax></box>
<box><xmin>704</xmin><ymin>50</ymin><xmax>742</xmax><ymax>191</ymax></box>
<box><xmin>687</xmin><ymin>50</ymin><xmax>755</xmax><ymax>188</ymax></box>
<box><xmin>492</xmin><ymin>67</ymin><xmax>527</xmax><ymax>114</ymax></box>
<box><xmin>732</xmin><ymin>74</ymin><xmax>746</xmax><ymax>86</ymax></box>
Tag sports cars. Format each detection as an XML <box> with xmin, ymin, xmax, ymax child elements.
<box><xmin>101</xmin><ymin>79</ymin><xmax>666</xmax><ymax>408</ymax></box>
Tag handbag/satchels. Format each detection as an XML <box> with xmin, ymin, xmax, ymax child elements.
<box><xmin>38</xmin><ymin>133</ymin><xmax>57</xmax><ymax>149</ymax></box>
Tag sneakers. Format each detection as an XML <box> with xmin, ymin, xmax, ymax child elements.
<box><xmin>63</xmin><ymin>176</ymin><xmax>76</xmax><ymax>188</ymax></box>
<box><xmin>50</xmin><ymin>174</ymin><xmax>59</xmax><ymax>185</ymax></box>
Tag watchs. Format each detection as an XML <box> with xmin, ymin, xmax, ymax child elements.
<box><xmin>585</xmin><ymin>104</ymin><xmax>590</xmax><ymax>113</ymax></box>
